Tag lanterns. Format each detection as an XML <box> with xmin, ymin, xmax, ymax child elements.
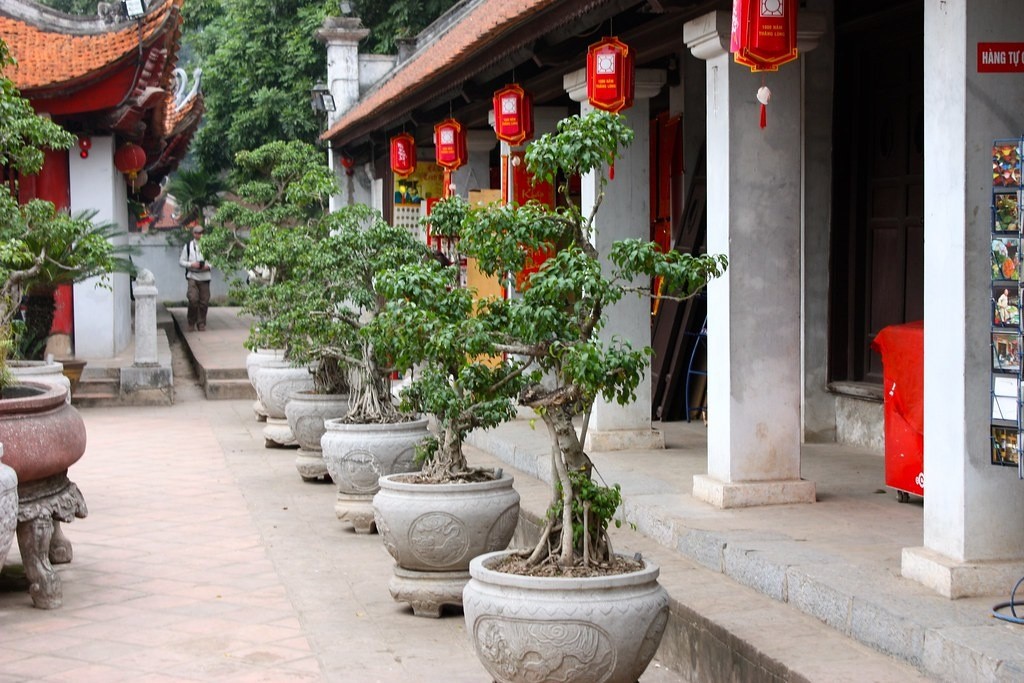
<box><xmin>434</xmin><ymin>118</ymin><xmax>468</xmax><ymax>194</ymax></box>
<box><xmin>114</xmin><ymin>141</ymin><xmax>161</xmax><ymax>205</ymax></box>
<box><xmin>730</xmin><ymin>0</ymin><xmax>798</xmax><ymax>129</ymax></box>
<box><xmin>587</xmin><ymin>36</ymin><xmax>636</xmax><ymax>181</ymax></box>
<box><xmin>390</xmin><ymin>133</ymin><xmax>417</xmax><ymax>205</ymax></box>
<box><xmin>494</xmin><ymin>83</ymin><xmax>535</xmax><ymax>180</ymax></box>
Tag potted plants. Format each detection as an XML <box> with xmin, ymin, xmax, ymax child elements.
<box><xmin>0</xmin><ymin>207</ymin><xmax>134</xmax><ymax>611</ymax></box>
<box><xmin>203</xmin><ymin>118</ymin><xmax>727</xmax><ymax>683</ymax></box>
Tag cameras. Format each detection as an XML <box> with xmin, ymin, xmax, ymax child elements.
<box><xmin>199</xmin><ymin>261</ymin><xmax>205</xmax><ymax>269</ymax></box>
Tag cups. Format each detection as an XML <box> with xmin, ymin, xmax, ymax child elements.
<box><xmin>200</xmin><ymin>261</ymin><xmax>204</xmax><ymax>268</ymax></box>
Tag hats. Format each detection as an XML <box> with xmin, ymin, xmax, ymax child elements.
<box><xmin>193</xmin><ymin>225</ymin><xmax>203</xmax><ymax>233</ymax></box>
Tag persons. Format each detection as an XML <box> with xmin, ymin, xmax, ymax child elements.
<box><xmin>997</xmin><ymin>289</ymin><xmax>1011</xmax><ymax>325</ymax></box>
<box><xmin>180</xmin><ymin>226</ymin><xmax>212</xmax><ymax>332</ymax></box>
<box><xmin>245</xmin><ymin>261</ymin><xmax>277</xmax><ymax>286</ymax></box>
<box><xmin>395</xmin><ymin>179</ymin><xmax>425</xmax><ymax>204</ymax></box>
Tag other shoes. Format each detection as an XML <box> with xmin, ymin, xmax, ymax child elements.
<box><xmin>189</xmin><ymin>322</ymin><xmax>206</xmax><ymax>332</ymax></box>
